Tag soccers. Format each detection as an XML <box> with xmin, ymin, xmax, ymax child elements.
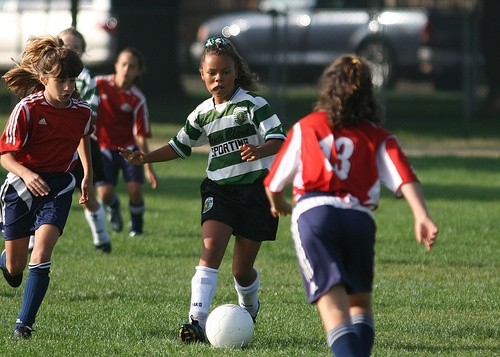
<box><xmin>205</xmin><ymin>303</ymin><xmax>254</xmax><ymax>349</ymax></box>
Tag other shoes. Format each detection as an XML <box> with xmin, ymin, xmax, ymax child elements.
<box><xmin>129</xmin><ymin>229</ymin><xmax>141</xmax><ymax>237</ymax></box>
<box><xmin>96</xmin><ymin>243</ymin><xmax>110</xmax><ymax>253</ymax></box>
<box><xmin>109</xmin><ymin>200</ymin><xmax>123</xmax><ymax>231</ymax></box>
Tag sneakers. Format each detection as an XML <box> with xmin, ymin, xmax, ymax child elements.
<box><xmin>246</xmin><ymin>301</ymin><xmax>259</xmax><ymax>324</ymax></box>
<box><xmin>12</xmin><ymin>327</ymin><xmax>35</xmax><ymax>342</ymax></box>
<box><xmin>179</xmin><ymin>316</ymin><xmax>206</xmax><ymax>341</ymax></box>
<box><xmin>0</xmin><ymin>249</ymin><xmax>23</xmax><ymax>287</ymax></box>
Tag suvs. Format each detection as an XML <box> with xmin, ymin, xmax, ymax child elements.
<box><xmin>0</xmin><ymin>0</ymin><xmax>167</xmax><ymax>92</ymax></box>
<box><xmin>182</xmin><ymin>0</ymin><xmax>473</xmax><ymax>92</ymax></box>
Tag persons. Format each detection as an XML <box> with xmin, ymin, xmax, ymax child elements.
<box><xmin>0</xmin><ymin>37</ymin><xmax>94</xmax><ymax>339</ymax></box>
<box><xmin>118</xmin><ymin>36</ymin><xmax>288</xmax><ymax>342</ymax></box>
<box><xmin>262</xmin><ymin>54</ymin><xmax>439</xmax><ymax>357</ymax></box>
<box><xmin>92</xmin><ymin>47</ymin><xmax>157</xmax><ymax>237</ymax></box>
<box><xmin>57</xmin><ymin>29</ymin><xmax>112</xmax><ymax>253</ymax></box>
